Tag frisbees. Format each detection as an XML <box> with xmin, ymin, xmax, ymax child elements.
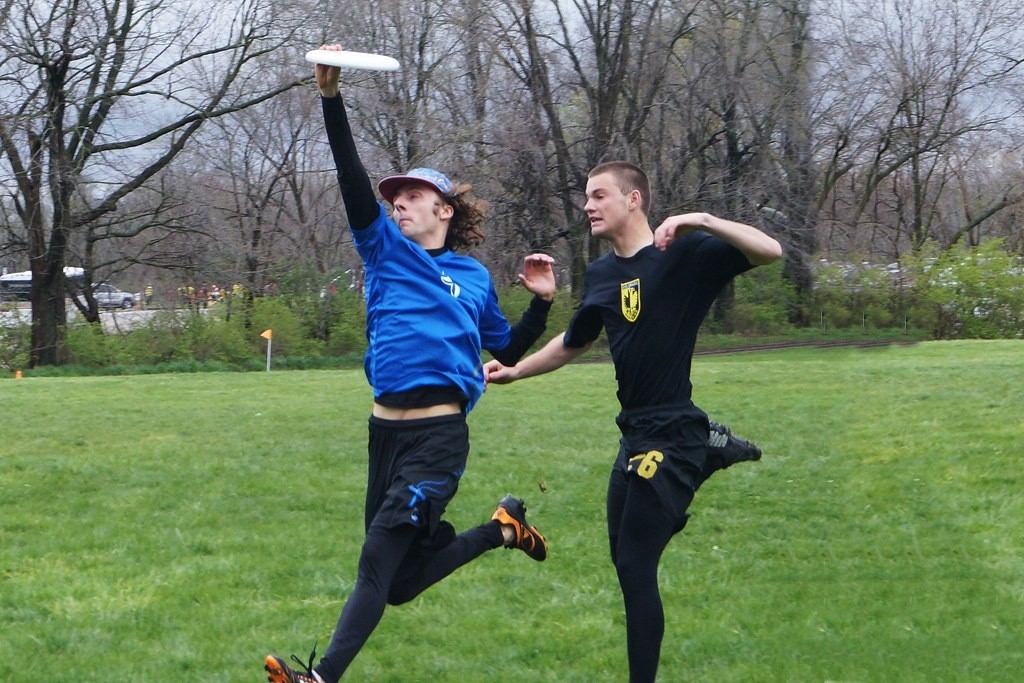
<box><xmin>304</xmin><ymin>46</ymin><xmax>402</xmax><ymax>73</ymax></box>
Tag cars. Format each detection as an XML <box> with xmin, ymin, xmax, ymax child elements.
<box><xmin>72</xmin><ymin>283</ymin><xmax>135</xmax><ymax>310</ymax></box>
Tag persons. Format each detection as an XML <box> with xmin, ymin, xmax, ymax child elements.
<box><xmin>176</xmin><ymin>281</ymin><xmax>245</xmax><ymax>308</ymax></box>
<box><xmin>482</xmin><ymin>162</ymin><xmax>782</xmax><ymax>683</ymax></box>
<box><xmin>145</xmin><ymin>284</ymin><xmax>153</xmax><ymax>303</ymax></box>
<box><xmin>264</xmin><ymin>44</ymin><xmax>557</xmax><ymax>683</ymax></box>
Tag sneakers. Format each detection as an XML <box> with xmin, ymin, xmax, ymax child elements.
<box><xmin>491</xmin><ymin>493</ymin><xmax>548</xmax><ymax>563</ymax></box>
<box><xmin>707</xmin><ymin>419</ymin><xmax>762</xmax><ymax>470</ymax></box>
<box><xmin>264</xmin><ymin>654</ymin><xmax>325</xmax><ymax>683</ymax></box>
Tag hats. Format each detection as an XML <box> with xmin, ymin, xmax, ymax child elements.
<box><xmin>377</xmin><ymin>167</ymin><xmax>455</xmax><ymax>203</ymax></box>
